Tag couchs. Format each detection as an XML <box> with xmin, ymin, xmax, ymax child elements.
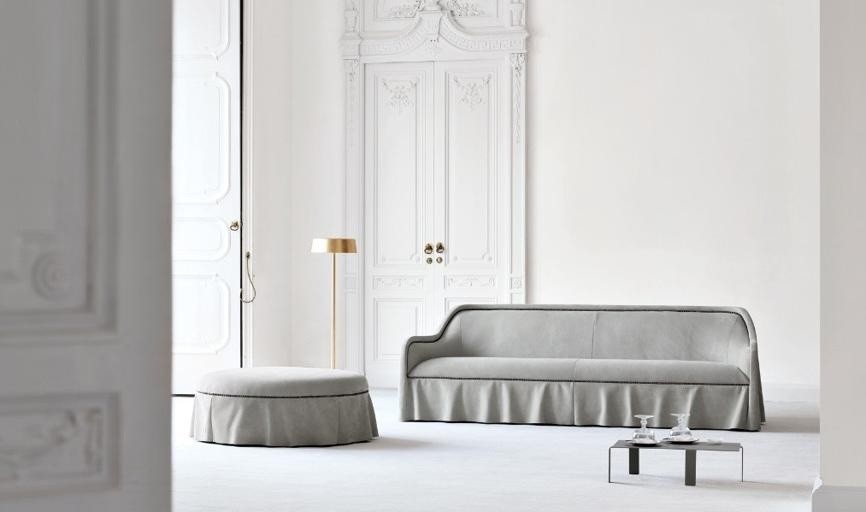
<box><xmin>399</xmin><ymin>303</ymin><xmax>766</xmax><ymax>431</ymax></box>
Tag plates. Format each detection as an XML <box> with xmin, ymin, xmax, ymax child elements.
<box><xmin>625</xmin><ymin>440</ymin><xmax>662</xmax><ymax>445</ymax></box>
<box><xmin>663</xmin><ymin>438</ymin><xmax>699</xmax><ymax>443</ymax></box>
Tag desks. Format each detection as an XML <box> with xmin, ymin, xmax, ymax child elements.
<box><xmin>609</xmin><ymin>439</ymin><xmax>744</xmax><ymax>486</ymax></box>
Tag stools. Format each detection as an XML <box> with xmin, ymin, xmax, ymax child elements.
<box><xmin>189</xmin><ymin>363</ymin><xmax>380</xmax><ymax>449</ymax></box>
<box><xmin>308</xmin><ymin>237</ymin><xmax>358</xmax><ymax>368</ymax></box>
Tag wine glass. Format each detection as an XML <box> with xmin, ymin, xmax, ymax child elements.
<box><xmin>632</xmin><ymin>414</ymin><xmax>655</xmax><ymax>444</ymax></box>
<box><xmin>669</xmin><ymin>413</ymin><xmax>693</xmax><ymax>440</ymax></box>
